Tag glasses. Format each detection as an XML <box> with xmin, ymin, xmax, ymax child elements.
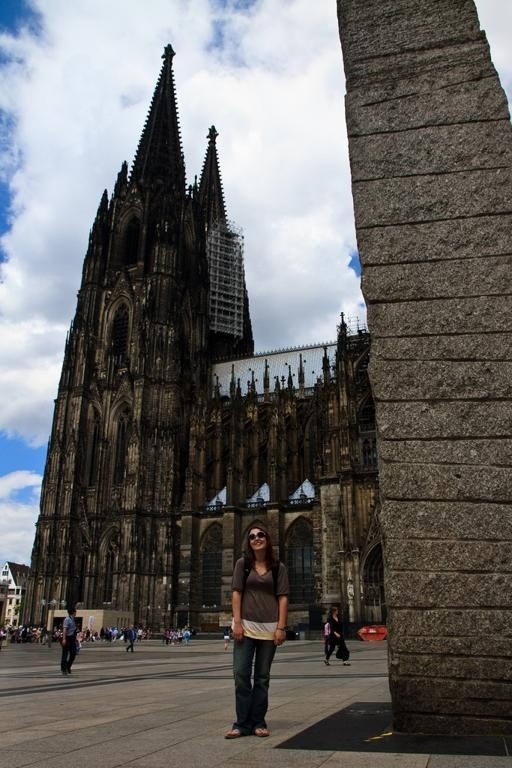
<box><xmin>248</xmin><ymin>531</ymin><xmax>268</xmax><ymax>540</ymax></box>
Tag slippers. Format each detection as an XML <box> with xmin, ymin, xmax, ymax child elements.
<box><xmin>255</xmin><ymin>727</ymin><xmax>269</xmax><ymax>737</ymax></box>
<box><xmin>225</xmin><ymin>729</ymin><xmax>241</xmax><ymax>739</ymax></box>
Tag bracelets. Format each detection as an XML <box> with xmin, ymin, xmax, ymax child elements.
<box><xmin>275</xmin><ymin>627</ymin><xmax>285</xmax><ymax>631</ymax></box>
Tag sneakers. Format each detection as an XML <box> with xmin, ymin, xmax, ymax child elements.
<box><xmin>344</xmin><ymin>661</ymin><xmax>350</xmax><ymax>666</ymax></box>
<box><xmin>324</xmin><ymin>659</ymin><xmax>329</xmax><ymax>665</ymax></box>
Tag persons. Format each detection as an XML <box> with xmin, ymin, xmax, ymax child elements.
<box><xmin>224</xmin><ymin>524</ymin><xmax>289</xmax><ymax>738</ymax></box>
<box><xmin>324</xmin><ymin>618</ymin><xmax>332</xmax><ymax>654</ymax></box>
<box><xmin>323</xmin><ymin>606</ymin><xmax>351</xmax><ymax>667</ymax></box>
<box><xmin>224</xmin><ymin>626</ymin><xmax>229</xmax><ymax>651</ymax></box>
<box><xmin>60</xmin><ymin>605</ymin><xmax>80</xmax><ymax>675</ymax></box>
<box><xmin>0</xmin><ymin>615</ymin><xmax>191</xmax><ymax>652</ymax></box>
